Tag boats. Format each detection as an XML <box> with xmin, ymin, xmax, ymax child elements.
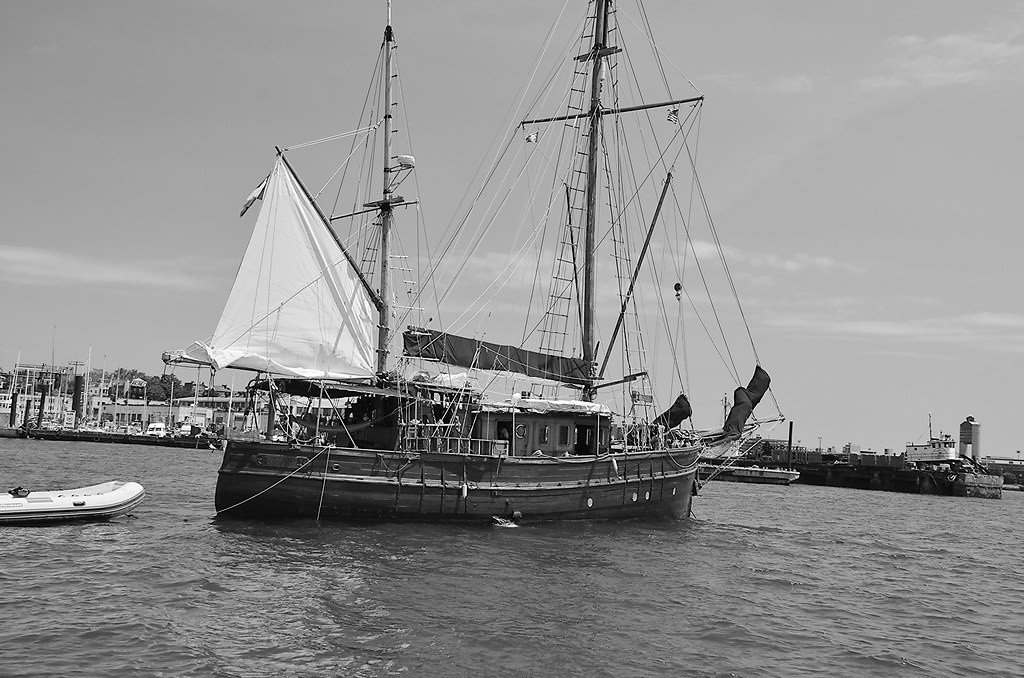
<box><xmin>906</xmin><ymin>413</ymin><xmax>975</xmax><ymax>472</ymax></box>
<box><xmin>697</xmin><ymin>462</ymin><xmax>801</xmax><ymax>485</ymax></box>
<box><xmin>0</xmin><ymin>478</ymin><xmax>146</xmax><ymax>522</ymax></box>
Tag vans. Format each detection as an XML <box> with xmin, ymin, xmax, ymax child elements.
<box><xmin>146</xmin><ymin>422</ymin><xmax>166</xmax><ymax>437</ymax></box>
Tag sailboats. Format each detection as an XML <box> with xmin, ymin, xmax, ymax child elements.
<box><xmin>158</xmin><ymin>0</ymin><xmax>788</xmax><ymax>528</ymax></box>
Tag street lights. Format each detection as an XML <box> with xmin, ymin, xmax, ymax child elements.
<box><xmin>818</xmin><ymin>436</ymin><xmax>823</xmax><ymax>464</ymax></box>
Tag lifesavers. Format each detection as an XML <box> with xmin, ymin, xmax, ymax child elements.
<box><xmin>515</xmin><ymin>424</ymin><xmax>527</xmax><ymax>439</ymax></box>
